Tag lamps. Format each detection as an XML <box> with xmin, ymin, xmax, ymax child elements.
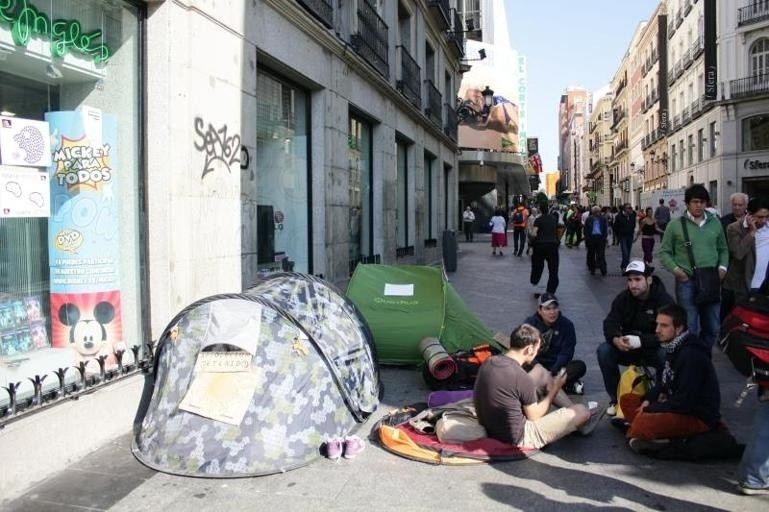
<box><xmin>630</xmin><ymin>150</ymin><xmax>661</xmax><ymax>173</ymax></box>
<box><xmin>456</xmin><ymin>86</ymin><xmax>493</xmax><ymax>117</ymax></box>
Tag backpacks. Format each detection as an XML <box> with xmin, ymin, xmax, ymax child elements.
<box><xmin>423</xmin><ymin>344</ymin><xmax>501</xmax><ymax>390</ymax></box>
<box><xmin>512</xmin><ymin>207</ymin><xmax>526</xmax><ymax>225</ymax></box>
<box><xmin>717</xmin><ymin>290</ymin><xmax>767</xmax><ymax>403</ymax></box>
<box><xmin>434</xmin><ymin>399</ymin><xmax>487</xmax><ymax>444</ymax></box>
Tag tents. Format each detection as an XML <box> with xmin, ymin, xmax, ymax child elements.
<box><xmin>346</xmin><ymin>262</ymin><xmax>508</xmax><ymax>368</ymax></box>
<box><xmin>131</xmin><ymin>273</ymin><xmax>385</xmax><ymax>479</ymax></box>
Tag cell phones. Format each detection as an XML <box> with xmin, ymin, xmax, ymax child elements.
<box><xmin>744</xmin><ymin>208</ymin><xmax>749</xmax><ymax>214</ymax></box>
<box><xmin>559</xmin><ymin>367</ymin><xmax>567</xmax><ymax>377</ymax></box>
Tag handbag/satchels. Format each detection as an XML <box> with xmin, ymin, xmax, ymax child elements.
<box><xmin>427</xmin><ymin>390</ymin><xmax>474</xmax><ymax>408</ymax></box>
<box><xmin>615</xmin><ymin>361</ymin><xmax>655</xmax><ymax>415</ymax></box>
<box><xmin>692</xmin><ymin>266</ymin><xmax>720</xmax><ymax>306</ymax></box>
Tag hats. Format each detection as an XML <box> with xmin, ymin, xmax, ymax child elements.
<box><xmin>569</xmin><ymin>200</ymin><xmax>577</xmax><ymax>206</ymax></box>
<box><xmin>622</xmin><ymin>260</ymin><xmax>650</xmax><ymax>278</ymax></box>
<box><xmin>538</xmin><ymin>293</ymin><xmax>560</xmax><ymax>307</ymax></box>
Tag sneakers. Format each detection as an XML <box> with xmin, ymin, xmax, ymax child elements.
<box><xmin>610</xmin><ymin>416</ymin><xmax>630</xmax><ymax>433</ymax></box>
<box><xmin>738</xmin><ymin>476</ymin><xmax>769</xmax><ymax>496</ymax></box>
<box><xmin>579</xmin><ymin>406</ymin><xmax>607</xmax><ymax>436</ymax></box>
<box><xmin>629</xmin><ymin>437</ymin><xmax>658</xmax><ymax>454</ymax></box>
<box><xmin>565</xmin><ymin>379</ymin><xmax>584</xmax><ymax>395</ymax></box>
<box><xmin>606</xmin><ymin>402</ymin><xmax>618</xmax><ymax>415</ymax></box>
<box><xmin>325</xmin><ymin>435</ymin><xmax>366</xmax><ymax>459</ymax></box>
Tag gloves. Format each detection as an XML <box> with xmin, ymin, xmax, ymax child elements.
<box><xmin>623</xmin><ymin>335</ymin><xmax>642</xmax><ymax>350</ymax></box>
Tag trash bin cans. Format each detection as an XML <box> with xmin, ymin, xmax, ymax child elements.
<box><xmin>443</xmin><ymin>229</ymin><xmax>457</xmax><ymax>272</ymax></box>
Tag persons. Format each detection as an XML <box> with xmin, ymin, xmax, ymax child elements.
<box><xmin>462</xmin><ymin>205</ymin><xmax>475</xmax><ymax>243</ymax></box>
<box><xmin>611</xmin><ymin>303</ymin><xmax>721</xmax><ymax>461</ymax></box>
<box><xmin>705</xmin><ymin>192</ymin><xmax>769</xmax><ymax>325</ymax></box>
<box><xmin>659</xmin><ymin>184</ymin><xmax>730</xmax><ymax>350</ymax></box>
<box><xmin>738</xmin><ymin>386</ymin><xmax>769</xmax><ymax>494</ymax></box>
<box><xmin>522</xmin><ymin>293</ymin><xmax>586</xmax><ymax>395</ymax></box>
<box><xmin>597</xmin><ymin>259</ymin><xmax>675</xmax><ymax>416</ymax></box>
<box><xmin>473</xmin><ymin>322</ymin><xmax>607</xmax><ymax>450</ymax></box>
<box><xmin>488</xmin><ymin>192</ymin><xmax>670</xmax><ymax>293</ymax></box>
